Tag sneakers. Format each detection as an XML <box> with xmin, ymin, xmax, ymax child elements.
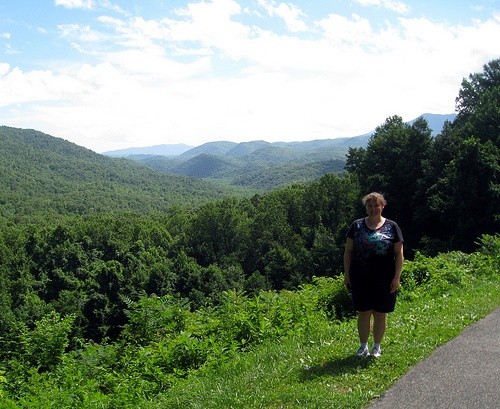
<box><xmin>356</xmin><ymin>346</ymin><xmax>370</xmax><ymax>357</ymax></box>
<box><xmin>370</xmin><ymin>346</ymin><xmax>381</xmax><ymax>357</ymax></box>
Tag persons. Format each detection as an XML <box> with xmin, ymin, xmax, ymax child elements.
<box><xmin>342</xmin><ymin>193</ymin><xmax>403</xmax><ymax>360</ymax></box>
